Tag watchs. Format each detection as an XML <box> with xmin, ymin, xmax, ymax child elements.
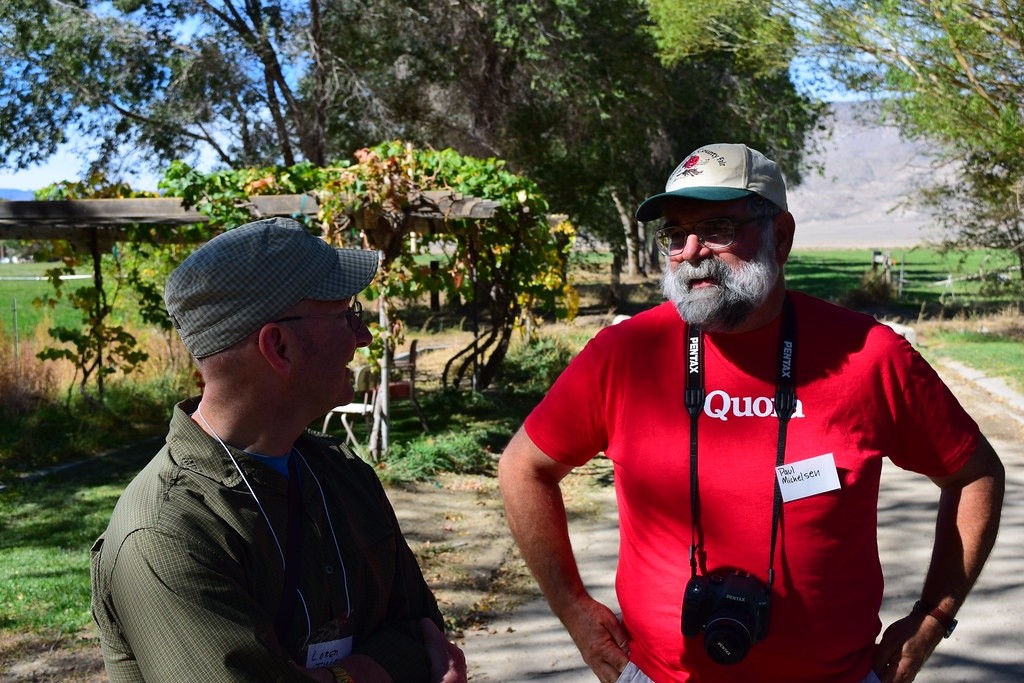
<box><xmin>912</xmin><ymin>601</ymin><xmax>958</xmax><ymax>639</ymax></box>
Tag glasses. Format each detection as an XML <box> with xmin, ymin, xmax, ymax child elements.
<box><xmin>255</xmin><ymin>302</ymin><xmax>362</xmax><ymax>345</ymax></box>
<box><xmin>655</xmin><ymin>217</ymin><xmax>773</xmax><ymax>254</ymax></box>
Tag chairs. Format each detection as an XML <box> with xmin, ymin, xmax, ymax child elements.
<box><xmin>321</xmin><ymin>365</ymin><xmax>378</xmax><ymax>457</ymax></box>
<box><xmin>359</xmin><ymin>340</ymin><xmax>433</xmax><ymax>436</ymax></box>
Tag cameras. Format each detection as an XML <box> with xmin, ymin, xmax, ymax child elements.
<box><xmin>681</xmin><ymin>568</ymin><xmax>772</xmax><ymax>665</ymax></box>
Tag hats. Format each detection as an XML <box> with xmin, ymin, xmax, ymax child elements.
<box><xmin>164</xmin><ymin>218</ymin><xmax>378</xmax><ymax>358</ymax></box>
<box><xmin>635</xmin><ymin>143</ymin><xmax>786</xmax><ymax>222</ymax></box>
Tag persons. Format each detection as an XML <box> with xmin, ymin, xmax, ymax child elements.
<box><xmin>499</xmin><ymin>142</ymin><xmax>1007</xmax><ymax>683</ymax></box>
<box><xmin>91</xmin><ymin>216</ymin><xmax>467</xmax><ymax>683</ymax></box>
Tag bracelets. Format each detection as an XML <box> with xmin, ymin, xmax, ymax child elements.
<box><xmin>323</xmin><ymin>664</ymin><xmax>354</xmax><ymax>683</ymax></box>
